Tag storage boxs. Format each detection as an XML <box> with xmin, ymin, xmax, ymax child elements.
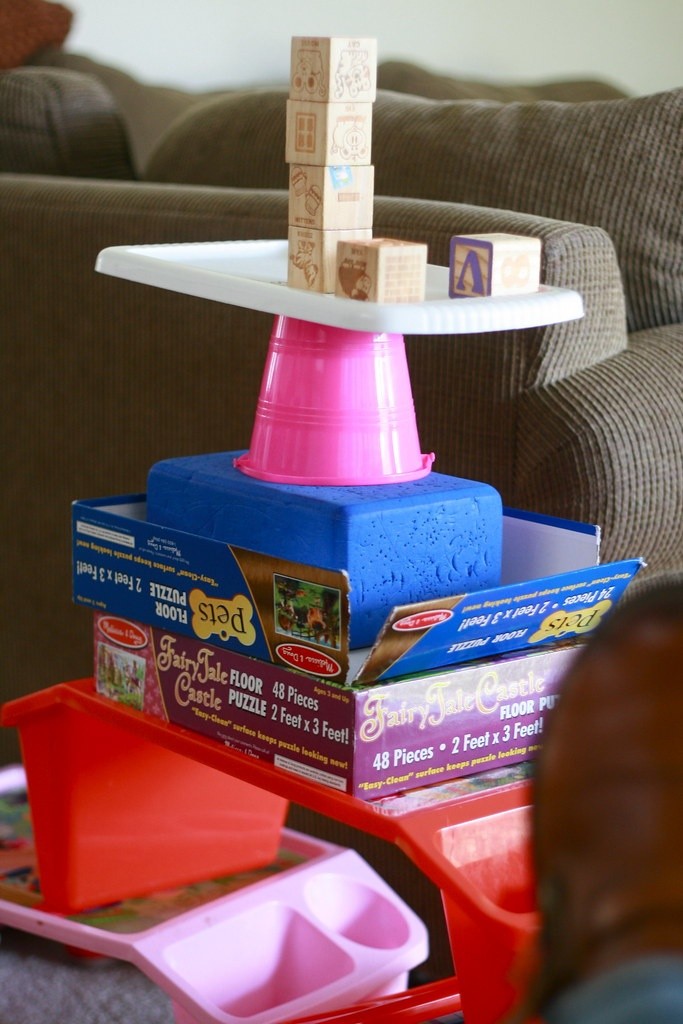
<box><xmin>93</xmin><ymin>609</ymin><xmax>593</xmax><ymax>799</ymax></box>
<box><xmin>68</xmin><ymin>494</ymin><xmax>647</xmax><ymax>689</ymax></box>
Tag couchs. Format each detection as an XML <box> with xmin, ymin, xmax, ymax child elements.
<box><xmin>0</xmin><ymin>47</ymin><xmax>683</xmax><ymax>764</ymax></box>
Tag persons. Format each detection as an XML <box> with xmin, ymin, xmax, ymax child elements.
<box><xmin>523</xmin><ymin>576</ymin><xmax>683</xmax><ymax>1023</ymax></box>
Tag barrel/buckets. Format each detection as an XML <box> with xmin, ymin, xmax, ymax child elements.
<box><xmin>234</xmin><ymin>314</ymin><xmax>436</xmax><ymax>488</ymax></box>
